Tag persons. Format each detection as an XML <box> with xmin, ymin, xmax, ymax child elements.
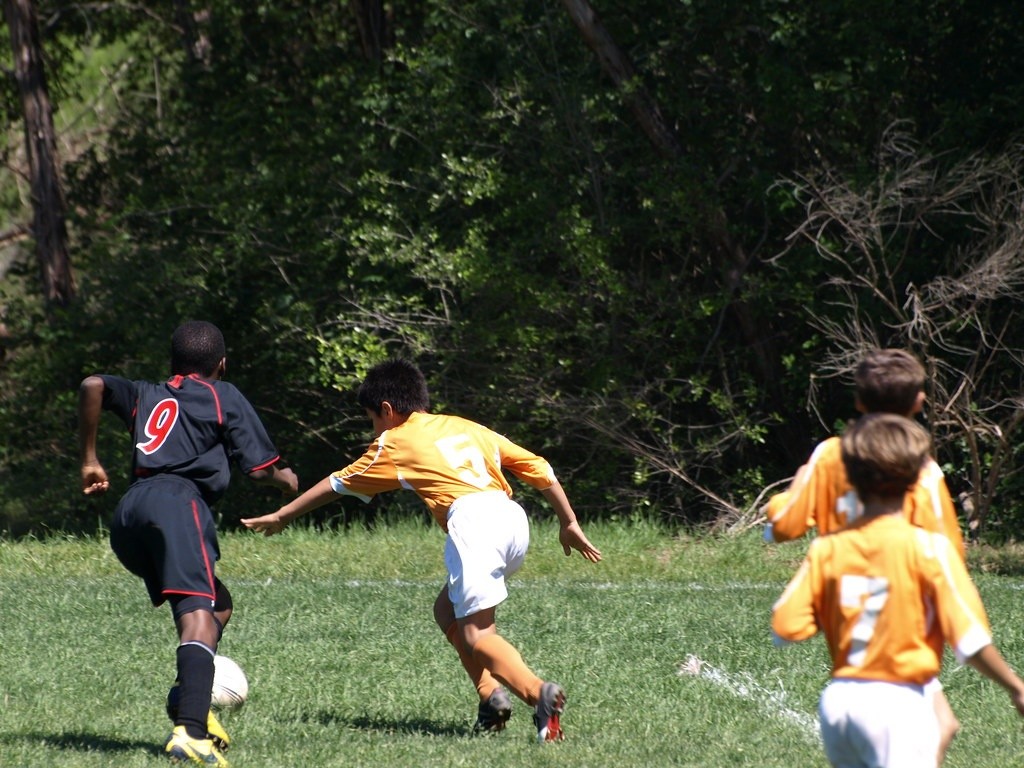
<box><xmin>772</xmin><ymin>412</ymin><xmax>1024</xmax><ymax>768</ymax></box>
<box><xmin>239</xmin><ymin>356</ymin><xmax>602</xmax><ymax>744</ymax></box>
<box><xmin>78</xmin><ymin>320</ymin><xmax>298</xmax><ymax>768</ymax></box>
<box><xmin>765</xmin><ymin>349</ymin><xmax>968</xmax><ymax>768</ymax></box>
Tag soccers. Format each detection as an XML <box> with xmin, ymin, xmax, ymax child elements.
<box><xmin>209</xmin><ymin>655</ymin><xmax>248</xmax><ymax>712</ymax></box>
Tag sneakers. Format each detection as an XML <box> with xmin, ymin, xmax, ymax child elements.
<box><xmin>470</xmin><ymin>687</ymin><xmax>512</xmax><ymax>738</ymax></box>
<box><xmin>531</xmin><ymin>682</ymin><xmax>566</xmax><ymax>742</ymax></box>
<box><xmin>166</xmin><ymin>725</ymin><xmax>232</xmax><ymax>768</ymax></box>
<box><xmin>166</xmin><ymin>686</ymin><xmax>229</xmax><ymax>752</ymax></box>
